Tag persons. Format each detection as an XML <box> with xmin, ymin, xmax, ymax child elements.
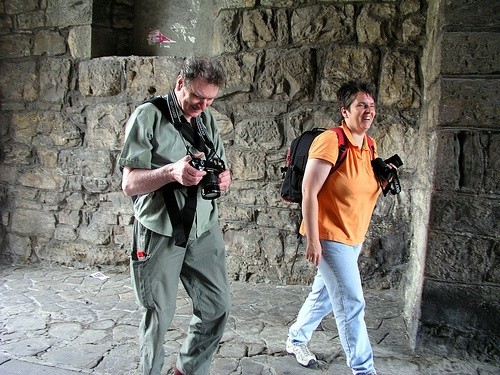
<box><xmin>121</xmin><ymin>57</ymin><xmax>233</xmax><ymax>375</ymax></box>
<box><xmin>286</xmin><ymin>80</ymin><xmax>397</xmax><ymax>375</ymax></box>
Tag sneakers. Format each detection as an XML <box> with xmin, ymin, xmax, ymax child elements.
<box><xmin>284</xmin><ymin>338</ymin><xmax>317</xmax><ymax>367</ymax></box>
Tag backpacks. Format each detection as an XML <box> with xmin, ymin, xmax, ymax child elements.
<box><xmin>278</xmin><ymin>127</ymin><xmax>376</xmax><ymax>206</ymax></box>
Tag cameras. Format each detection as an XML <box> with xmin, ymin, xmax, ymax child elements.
<box><xmin>189</xmin><ymin>157</ymin><xmax>226</xmax><ymax>200</ymax></box>
<box><xmin>371</xmin><ymin>153</ymin><xmax>403</xmax><ymax>182</ymax></box>
